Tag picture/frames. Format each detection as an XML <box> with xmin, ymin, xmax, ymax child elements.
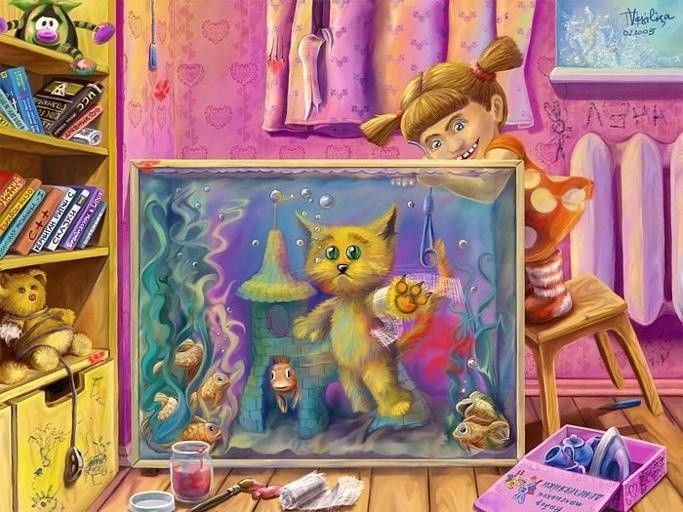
<box><xmin>129</xmin><ymin>159</ymin><xmax>525</xmax><ymax>472</ymax></box>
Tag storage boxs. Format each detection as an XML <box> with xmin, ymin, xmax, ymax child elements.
<box><xmin>471</xmin><ymin>421</ymin><xmax>668</xmax><ymax>512</ymax></box>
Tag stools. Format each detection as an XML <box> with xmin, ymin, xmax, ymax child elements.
<box><xmin>524</xmin><ymin>272</ymin><xmax>664</xmax><ymax>442</ymax></box>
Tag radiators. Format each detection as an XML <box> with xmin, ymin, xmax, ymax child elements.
<box><xmin>570</xmin><ymin>131</ymin><xmax>682</xmax><ymax>322</ymax></box>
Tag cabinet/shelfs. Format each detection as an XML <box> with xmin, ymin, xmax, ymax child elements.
<box><xmin>0</xmin><ymin>0</ymin><xmax>118</xmax><ymax>512</ymax></box>
